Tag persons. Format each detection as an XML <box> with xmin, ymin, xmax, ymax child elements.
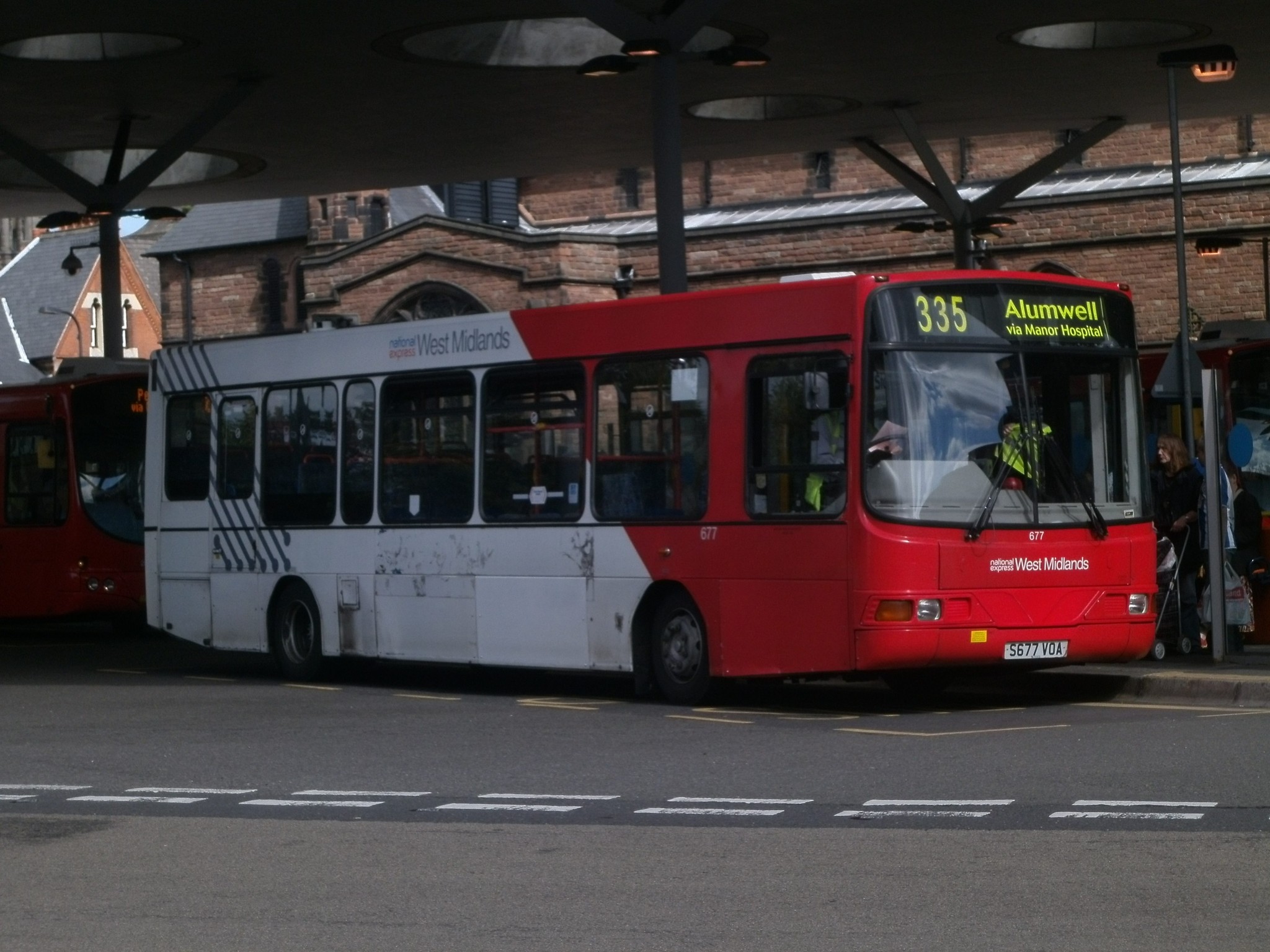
<box><xmin>994</xmin><ymin>376</ymin><xmax>1094</xmax><ymax>504</ymax></box>
<box><xmin>1149</xmin><ymin>432</ymin><xmax>1263</xmax><ymax>654</ymax></box>
<box><xmin>806</xmin><ymin>374</ymin><xmax>880</xmax><ymax>513</ymax></box>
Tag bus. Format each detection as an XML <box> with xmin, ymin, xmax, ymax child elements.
<box><xmin>145</xmin><ymin>271</ymin><xmax>1156</xmax><ymax>708</ymax></box>
<box><xmin>0</xmin><ymin>357</ymin><xmax>147</xmax><ymax>621</ymax></box>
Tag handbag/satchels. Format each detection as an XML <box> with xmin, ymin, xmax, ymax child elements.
<box><xmin>1201</xmin><ymin>560</ymin><xmax>1251</xmax><ymax>625</ymax></box>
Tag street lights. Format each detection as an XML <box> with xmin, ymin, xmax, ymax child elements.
<box><xmin>36</xmin><ymin>203</ymin><xmax>187</xmax><ymax>359</ymax></box>
<box><xmin>40</xmin><ymin>306</ymin><xmax>83</xmax><ymax>357</ymax></box>
<box><xmin>1195</xmin><ymin>237</ymin><xmax>1270</xmax><ymax>321</ymax></box>
<box><xmin>1159</xmin><ymin>44</ymin><xmax>1237</xmax><ymax>466</ymax></box>
<box><xmin>891</xmin><ymin>215</ymin><xmax>1018</xmax><ymax>270</ymax></box>
<box><xmin>577</xmin><ymin>38</ymin><xmax>771</xmax><ymax>294</ymax></box>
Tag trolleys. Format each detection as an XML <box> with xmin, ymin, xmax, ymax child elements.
<box><xmin>1151</xmin><ymin>523</ymin><xmax>1192</xmax><ymax>661</ymax></box>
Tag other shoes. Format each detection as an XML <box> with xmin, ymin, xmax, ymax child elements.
<box><xmin>1201</xmin><ymin>642</ymin><xmax>1213</xmax><ymax>654</ymax></box>
<box><xmin>1227</xmin><ymin>644</ymin><xmax>1244</xmax><ymax>655</ymax></box>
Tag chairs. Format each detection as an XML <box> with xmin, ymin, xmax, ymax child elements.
<box><xmin>201</xmin><ymin>445</ymin><xmax>659</xmax><ymax>530</ymax></box>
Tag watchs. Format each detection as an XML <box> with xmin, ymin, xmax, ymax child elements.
<box><xmin>1184</xmin><ymin>513</ymin><xmax>1191</xmax><ymax>525</ymax></box>
